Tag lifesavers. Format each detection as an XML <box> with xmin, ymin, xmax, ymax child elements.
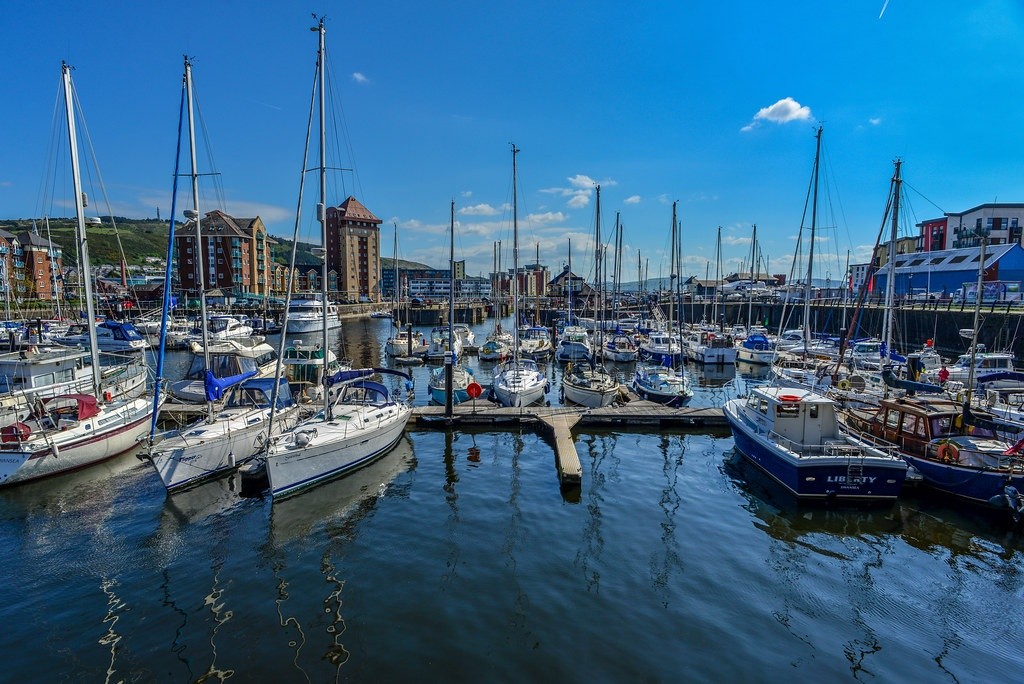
<box><xmin>467</xmin><ymin>382</ymin><xmax>482</xmax><ymax>399</ymax></box>
<box><xmin>708</xmin><ymin>334</ymin><xmax>716</xmax><ymax>340</ymax></box>
<box><xmin>838</xmin><ymin>379</ymin><xmax>850</xmax><ymax>390</ymax></box>
<box><xmin>780</xmin><ymin>394</ymin><xmax>802</xmax><ymax>401</ymax></box>
<box><xmin>937</xmin><ymin>443</ymin><xmax>960</xmax><ymax>464</ymax></box>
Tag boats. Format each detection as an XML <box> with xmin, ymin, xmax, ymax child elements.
<box><xmin>721</xmin><ymin>360</ymin><xmax>907</xmax><ymax>499</ymax></box>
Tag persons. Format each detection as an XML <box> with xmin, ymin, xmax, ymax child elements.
<box><xmin>938</xmin><ymin>366</ymin><xmax>949</xmax><ymax>386</ymax></box>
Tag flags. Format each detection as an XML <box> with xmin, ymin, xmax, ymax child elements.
<box><xmin>868</xmin><ymin>272</ymin><xmax>873</xmax><ymax>292</ymax></box>
<box><xmin>849</xmin><ymin>276</ymin><xmax>853</xmax><ymax>291</ymax></box>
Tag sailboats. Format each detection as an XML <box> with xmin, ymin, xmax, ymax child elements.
<box><xmin>553</xmin><ymin>122</ymin><xmax>1024</xmax><ymax>520</ymax></box>
<box><xmin>0</xmin><ymin>15</ymin><xmax>552</xmax><ymax>500</ymax></box>
